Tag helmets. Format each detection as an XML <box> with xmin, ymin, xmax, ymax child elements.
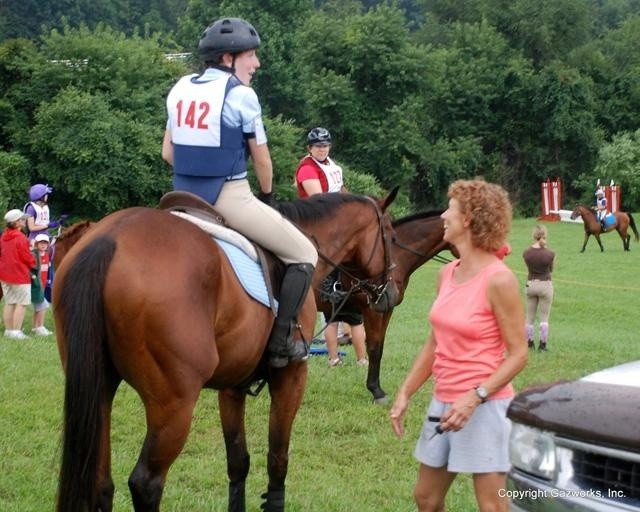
<box><xmin>29</xmin><ymin>184</ymin><xmax>52</xmax><ymax>200</ymax></box>
<box><xmin>308</xmin><ymin>126</ymin><xmax>330</xmax><ymax>146</ymax></box>
<box><xmin>195</xmin><ymin>15</ymin><xmax>261</xmax><ymax>60</ymax></box>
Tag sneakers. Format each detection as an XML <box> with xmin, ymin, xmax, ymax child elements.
<box><xmin>326</xmin><ymin>357</ymin><xmax>368</xmax><ymax>367</ymax></box>
<box><xmin>3</xmin><ymin>327</ymin><xmax>54</xmax><ymax>339</ymax></box>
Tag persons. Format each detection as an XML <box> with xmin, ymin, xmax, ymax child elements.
<box><xmin>23</xmin><ymin>184</ymin><xmax>69</xmax><ymax>307</ymax></box>
<box><xmin>28</xmin><ymin>233</ymin><xmax>53</xmax><ymax>336</ymax></box>
<box><xmin>490</xmin><ymin>242</ymin><xmax>512</xmax><ymax>261</ymax></box>
<box><xmin>522</xmin><ymin>225</ymin><xmax>554</xmax><ymax>351</ymax></box>
<box><xmin>591</xmin><ymin>189</ymin><xmax>607</xmax><ymax>232</ymax></box>
<box><xmin>390</xmin><ymin>177</ymin><xmax>529</xmax><ymax>512</ymax></box>
<box><xmin>324</xmin><ymin>303</ymin><xmax>369</xmax><ymax>368</ymax></box>
<box><xmin>162</xmin><ymin>16</ymin><xmax>318</xmax><ymax>370</ymax></box>
<box><xmin>0</xmin><ymin>209</ymin><xmax>37</xmax><ymax>340</ymax></box>
<box><xmin>296</xmin><ymin>128</ymin><xmax>349</xmax><ymax>202</ymax></box>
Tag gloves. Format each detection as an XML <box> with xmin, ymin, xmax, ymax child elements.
<box><xmin>49</xmin><ymin>215</ymin><xmax>68</xmax><ymax>228</ymax></box>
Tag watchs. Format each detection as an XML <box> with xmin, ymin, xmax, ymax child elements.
<box><xmin>473</xmin><ymin>386</ymin><xmax>487</xmax><ymax>404</ymax></box>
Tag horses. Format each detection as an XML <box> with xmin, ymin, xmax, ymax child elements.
<box><xmin>570</xmin><ymin>203</ymin><xmax>639</xmax><ymax>253</ymax></box>
<box><xmin>0</xmin><ymin>218</ymin><xmax>96</xmax><ymax>300</ymax></box>
<box><xmin>312</xmin><ymin>208</ymin><xmax>460</xmax><ymax>405</ymax></box>
<box><xmin>52</xmin><ymin>183</ymin><xmax>402</xmax><ymax>512</ymax></box>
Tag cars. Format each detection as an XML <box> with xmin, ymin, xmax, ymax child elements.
<box><xmin>505</xmin><ymin>357</ymin><xmax>639</xmax><ymax>511</ymax></box>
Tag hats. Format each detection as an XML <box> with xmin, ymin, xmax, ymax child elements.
<box><xmin>4</xmin><ymin>209</ymin><xmax>31</xmax><ymax>223</ymax></box>
<box><xmin>595</xmin><ymin>189</ymin><xmax>604</xmax><ymax>195</ymax></box>
<box><xmin>34</xmin><ymin>234</ymin><xmax>50</xmax><ymax>248</ymax></box>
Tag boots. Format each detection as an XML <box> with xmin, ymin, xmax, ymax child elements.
<box><xmin>527</xmin><ymin>339</ymin><xmax>549</xmax><ymax>351</ymax></box>
<box><xmin>268</xmin><ymin>263</ymin><xmax>309</xmax><ymax>367</ymax></box>
